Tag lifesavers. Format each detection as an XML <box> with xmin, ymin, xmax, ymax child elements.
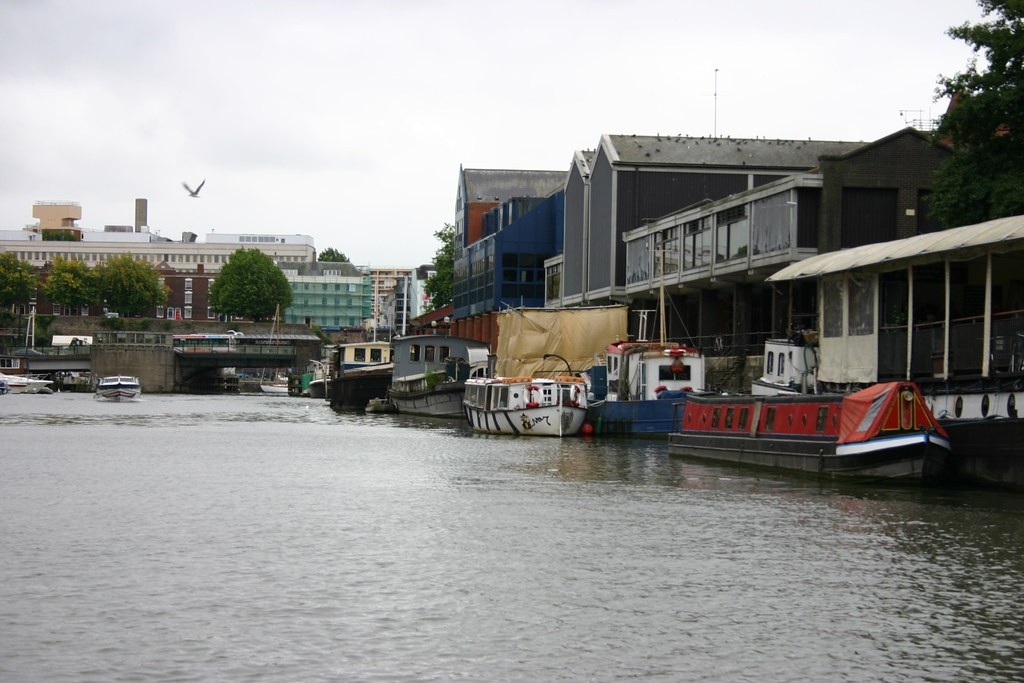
<box><xmin>569</xmin><ymin>385</ymin><xmax>582</xmax><ymax>407</ymax></box>
<box><xmin>663</xmin><ymin>348</ymin><xmax>687</xmax><ymax>358</ymax></box>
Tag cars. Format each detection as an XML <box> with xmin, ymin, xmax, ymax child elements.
<box><xmin>371</xmin><ymin>325</ymin><xmax>395</xmax><ymax>333</ymax></box>
<box><xmin>342</xmin><ymin>326</ymin><xmax>367</xmax><ymax>334</ymax></box>
<box><xmin>320</xmin><ymin>324</ymin><xmax>341</xmax><ymax>333</ymax></box>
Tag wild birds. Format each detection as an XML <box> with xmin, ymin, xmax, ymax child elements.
<box><xmin>182</xmin><ymin>179</ymin><xmax>205</xmax><ymax>198</ymax></box>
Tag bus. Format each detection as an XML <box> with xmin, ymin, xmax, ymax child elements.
<box><xmin>172</xmin><ymin>334</ymin><xmax>236</xmax><ymax>354</ymax></box>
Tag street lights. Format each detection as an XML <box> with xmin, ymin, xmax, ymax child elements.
<box><xmin>291</xmin><ymin>306</ymin><xmax>293</xmax><ymax>325</ymax></box>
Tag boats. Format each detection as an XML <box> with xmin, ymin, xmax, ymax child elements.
<box><xmin>96</xmin><ymin>375</ymin><xmax>142</xmax><ymax>399</ymax></box>
<box><xmin>463</xmin><ymin>375</ymin><xmax>587</xmax><ymax>438</ymax></box>
<box><xmin>302</xmin><ymin>340</ymin><xmax>393</xmax><ymax>414</ymax></box>
<box><xmin>387</xmin><ymin>334</ymin><xmax>496</xmax><ymax>418</ymax></box>
<box><xmin>668</xmin><ymin>330</ymin><xmax>954</xmax><ymax>486</ymax></box>
<box><xmin>0</xmin><ymin>372</ymin><xmax>54</xmax><ymax>394</ymax></box>
<box><xmin>575</xmin><ymin>308</ymin><xmax>716</xmax><ymax>438</ymax></box>
